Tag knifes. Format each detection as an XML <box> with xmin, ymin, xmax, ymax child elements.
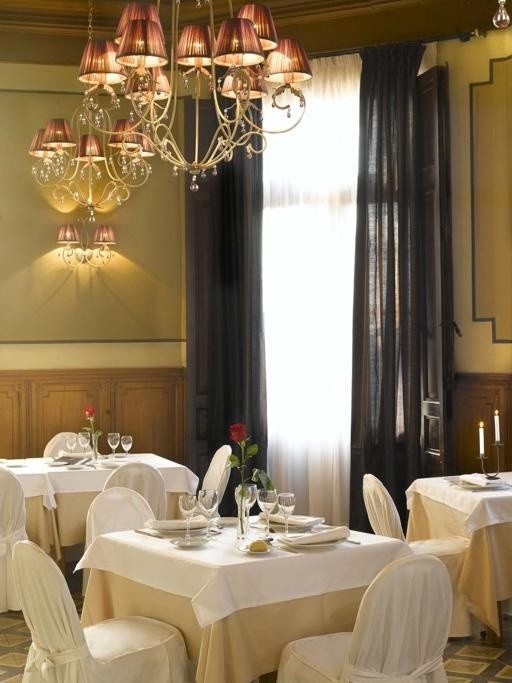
<box><xmin>135</xmin><ymin>529</ymin><xmax>164</xmax><ymax>539</ymax></box>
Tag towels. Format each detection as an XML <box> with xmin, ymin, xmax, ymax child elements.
<box><xmin>262</xmin><ymin>512</ymin><xmax>324</xmax><ymax>527</ymax></box>
<box><xmin>460</xmin><ymin>472</ymin><xmax>506</xmax><ymax>488</ymax></box>
<box><xmin>144</xmin><ymin>516</ymin><xmax>206</xmax><ymax>529</ymax></box>
<box><xmin>278</xmin><ymin>526</ymin><xmax>351</xmax><ymax>544</ymax></box>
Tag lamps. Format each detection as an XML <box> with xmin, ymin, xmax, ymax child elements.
<box><xmin>29</xmin><ymin>1</ymin><xmax>156</xmax><ymax>224</ymax></box>
<box><xmin>78</xmin><ymin>1</ymin><xmax>313</xmax><ymax>193</ymax></box>
<box><xmin>54</xmin><ymin>218</ymin><xmax>118</xmax><ymax>269</ymax></box>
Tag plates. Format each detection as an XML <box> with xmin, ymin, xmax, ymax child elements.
<box><xmin>168</xmin><ymin>537</ymin><xmax>210</xmax><ymax>548</ymax></box>
<box><xmin>157</xmin><ymin>528</ymin><xmax>209</xmax><ymax>537</ymax></box>
<box><xmin>238</xmin><ymin>538</ymin><xmax>276</xmax><ymax>554</ymax></box>
<box><xmin>283</xmin><ymin>533</ymin><xmax>347</xmax><ymax>549</ymax></box>
<box><xmin>257</xmin><ymin>517</ymin><xmax>313</xmax><ymax>532</ymax></box>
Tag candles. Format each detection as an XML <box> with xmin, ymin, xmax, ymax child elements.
<box><xmin>478</xmin><ymin>422</ymin><xmax>486</xmax><ymax>454</ymax></box>
<box><xmin>494</xmin><ymin>410</ymin><xmax>501</xmax><ymax>441</ymax></box>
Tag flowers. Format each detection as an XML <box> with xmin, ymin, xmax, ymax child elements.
<box><xmin>81</xmin><ymin>405</ymin><xmax>104</xmax><ymax>459</ymax></box>
<box><xmin>225</xmin><ymin>420</ymin><xmax>274</xmax><ymax>533</ymax></box>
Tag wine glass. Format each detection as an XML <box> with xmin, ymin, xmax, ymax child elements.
<box><xmin>277</xmin><ymin>493</ymin><xmax>295</xmax><ymax>539</ymax></box>
<box><xmin>66</xmin><ymin>433</ymin><xmax>77</xmax><ymax>453</ymax></box>
<box><xmin>107</xmin><ymin>432</ymin><xmax>120</xmax><ymax>461</ymax></box>
<box><xmin>178</xmin><ymin>492</ymin><xmax>198</xmax><ymax>546</ymax></box>
<box><xmin>238</xmin><ymin>483</ymin><xmax>257</xmax><ymax>537</ymax></box>
<box><xmin>121</xmin><ymin>435</ymin><xmax>133</xmax><ymax>461</ymax></box>
<box><xmin>198</xmin><ymin>489</ymin><xmax>219</xmax><ymax>540</ymax></box>
<box><xmin>256</xmin><ymin>488</ymin><xmax>278</xmax><ymax>540</ymax></box>
<box><xmin>79</xmin><ymin>431</ymin><xmax>91</xmax><ymax>453</ymax></box>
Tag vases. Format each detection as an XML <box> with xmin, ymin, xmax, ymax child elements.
<box><xmin>91</xmin><ymin>433</ymin><xmax>98</xmax><ymax>460</ymax></box>
<box><xmin>234</xmin><ymin>486</ymin><xmax>250</xmax><ymax>538</ymax></box>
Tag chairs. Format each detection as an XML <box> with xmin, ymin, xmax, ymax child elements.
<box><xmin>43</xmin><ymin>431</ymin><xmax>97</xmax><ymax>456</ymax></box>
<box><xmin>0</xmin><ymin>467</ymin><xmax>27</xmax><ymax>615</ymax></box>
<box><xmin>277</xmin><ymin>554</ymin><xmax>452</xmax><ymax>683</ymax></box>
<box><xmin>362</xmin><ymin>473</ymin><xmax>474</xmax><ymax>639</ymax></box>
<box><xmin>196</xmin><ymin>444</ymin><xmax>234</xmax><ymax>514</ymax></box>
<box><xmin>11</xmin><ymin>539</ymin><xmax>191</xmax><ymax>683</ymax></box>
<box><xmin>82</xmin><ymin>461</ymin><xmax>168</xmax><ymax>596</ymax></box>
<box><xmin>84</xmin><ymin>488</ymin><xmax>158</xmax><ymax>548</ymax></box>
